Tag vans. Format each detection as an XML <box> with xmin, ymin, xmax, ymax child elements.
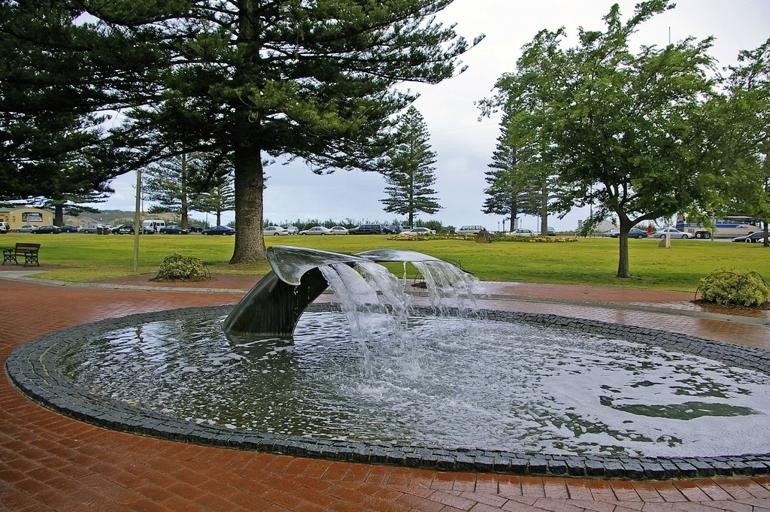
<box><xmin>455</xmin><ymin>225</ymin><xmax>483</xmax><ymax>234</ymax></box>
<box><xmin>142</xmin><ymin>220</ymin><xmax>166</xmax><ymax>234</ymax></box>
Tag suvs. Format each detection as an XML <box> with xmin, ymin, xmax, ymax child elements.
<box><xmin>0</xmin><ymin>222</ymin><xmax>11</xmax><ymax>233</ymax></box>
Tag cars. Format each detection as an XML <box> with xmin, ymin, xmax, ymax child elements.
<box><xmin>506</xmin><ymin>229</ymin><xmax>538</xmax><ymax>238</ymax></box>
<box><xmin>264</xmin><ymin>224</ymin><xmax>436</xmax><ymax>236</ymax></box>
<box><xmin>653</xmin><ymin>227</ymin><xmax>692</xmax><ymax>240</ymax></box>
<box><xmin>608</xmin><ymin>228</ymin><xmax>648</xmax><ymax>239</ymax></box>
<box><xmin>547</xmin><ymin>227</ymin><xmax>556</xmax><ymax>236</ymax></box>
<box><xmin>202</xmin><ymin>226</ymin><xmax>235</xmax><ymax>236</ymax></box>
<box><xmin>15</xmin><ymin>224</ymin><xmax>205</xmax><ymax>234</ymax></box>
<box><xmin>731</xmin><ymin>231</ymin><xmax>770</xmax><ymax>242</ymax></box>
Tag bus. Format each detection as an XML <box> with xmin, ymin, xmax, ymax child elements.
<box><xmin>676</xmin><ymin>212</ymin><xmax>761</xmax><ymax>239</ymax></box>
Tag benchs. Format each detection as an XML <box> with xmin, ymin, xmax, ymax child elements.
<box><xmin>2</xmin><ymin>242</ymin><xmax>41</xmax><ymax>267</ymax></box>
